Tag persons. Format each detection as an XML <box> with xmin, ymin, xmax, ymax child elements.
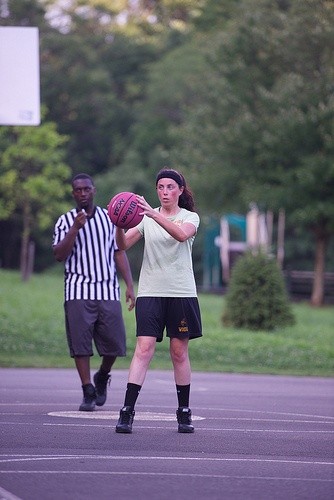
<box><xmin>107</xmin><ymin>170</ymin><xmax>202</xmax><ymax>433</ymax></box>
<box><xmin>52</xmin><ymin>173</ymin><xmax>136</xmax><ymax>411</ymax></box>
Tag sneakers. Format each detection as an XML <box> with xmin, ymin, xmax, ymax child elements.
<box><xmin>116</xmin><ymin>406</ymin><xmax>135</xmax><ymax>433</ymax></box>
<box><xmin>79</xmin><ymin>383</ymin><xmax>96</xmax><ymax>411</ymax></box>
<box><xmin>175</xmin><ymin>406</ymin><xmax>196</xmax><ymax>433</ymax></box>
<box><xmin>93</xmin><ymin>370</ymin><xmax>111</xmax><ymax>407</ymax></box>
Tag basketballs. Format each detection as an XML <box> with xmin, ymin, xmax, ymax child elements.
<box><xmin>108</xmin><ymin>191</ymin><xmax>146</xmax><ymax>229</ymax></box>
